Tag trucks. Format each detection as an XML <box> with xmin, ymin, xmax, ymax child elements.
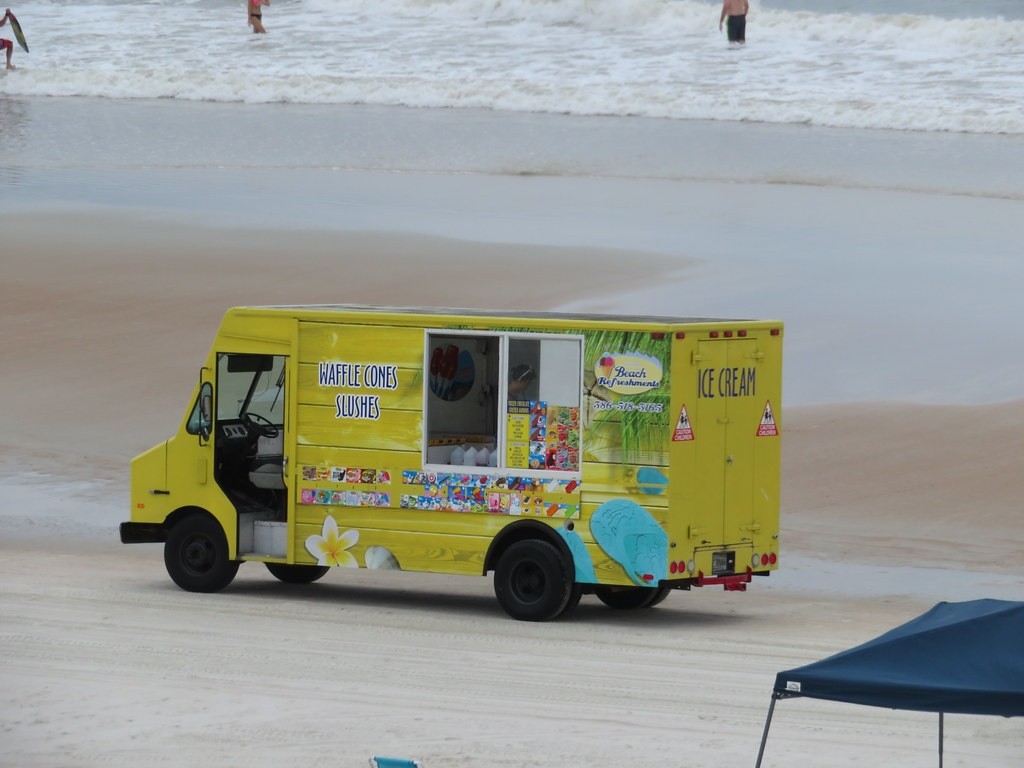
<box><xmin>119</xmin><ymin>304</ymin><xmax>785</xmax><ymax>622</ymax></box>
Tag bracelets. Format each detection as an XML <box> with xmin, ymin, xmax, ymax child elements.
<box><xmin>6</xmin><ymin>14</ymin><xmax>8</xmax><ymax>16</ymax></box>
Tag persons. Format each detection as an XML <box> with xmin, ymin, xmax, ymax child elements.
<box><xmin>0</xmin><ymin>8</ymin><xmax>16</xmax><ymax>69</ymax></box>
<box><xmin>508</xmin><ymin>364</ymin><xmax>535</xmax><ymax>400</ymax></box>
<box><xmin>720</xmin><ymin>0</ymin><xmax>749</xmax><ymax>44</ymax></box>
<box><xmin>247</xmin><ymin>0</ymin><xmax>269</xmax><ymax>33</ymax></box>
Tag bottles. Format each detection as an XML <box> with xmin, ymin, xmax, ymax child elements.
<box><xmin>464</xmin><ymin>446</ymin><xmax>478</xmax><ymax>466</ymax></box>
<box><xmin>489</xmin><ymin>448</ymin><xmax>497</xmax><ymax>467</ymax></box>
<box><xmin>450</xmin><ymin>444</ymin><xmax>465</xmax><ymax>465</ymax></box>
<box><xmin>476</xmin><ymin>445</ymin><xmax>490</xmax><ymax>466</ymax></box>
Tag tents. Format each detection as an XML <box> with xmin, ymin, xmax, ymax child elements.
<box><xmin>755</xmin><ymin>599</ymin><xmax>1024</xmax><ymax>768</ymax></box>
